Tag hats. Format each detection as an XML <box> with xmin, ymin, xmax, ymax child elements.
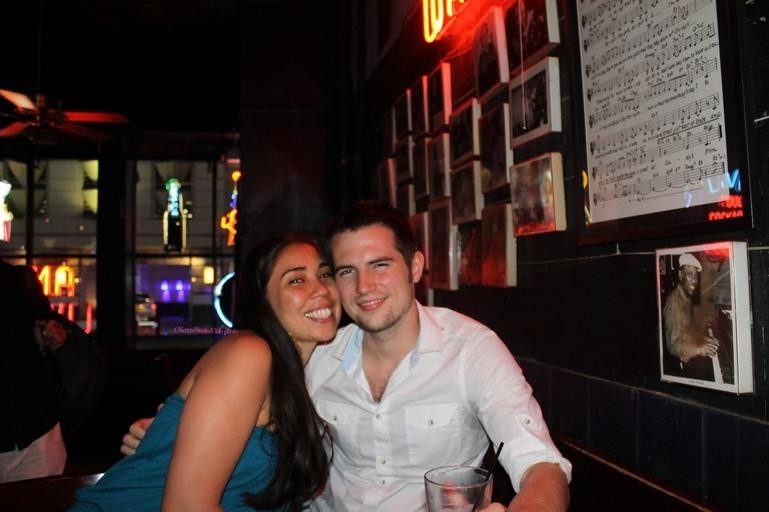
<box><xmin>678</xmin><ymin>253</ymin><xmax>703</xmax><ymax>272</ymax></box>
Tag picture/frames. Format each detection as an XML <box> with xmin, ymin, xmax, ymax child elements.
<box><xmin>427</xmin><ymin>198</ymin><xmax>460</xmax><ymax>292</ymax></box>
<box><xmin>508</xmin><ymin>56</ymin><xmax>563</xmax><ymax>150</ymax></box>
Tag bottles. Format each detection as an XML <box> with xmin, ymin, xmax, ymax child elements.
<box><xmin>162</xmin><ymin>178</ymin><xmax>185</xmax><ymax>252</ymax></box>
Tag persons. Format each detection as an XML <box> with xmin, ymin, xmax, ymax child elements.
<box><xmin>120</xmin><ymin>201</ymin><xmax>573</xmax><ymax>512</ymax></box>
<box><xmin>0</xmin><ymin>256</ymin><xmax>86</xmax><ymax>512</ymax></box>
<box><xmin>663</xmin><ymin>255</ymin><xmax>727</xmax><ymax>384</ymax></box>
<box><xmin>66</xmin><ymin>234</ymin><xmax>342</xmax><ymax>512</ymax></box>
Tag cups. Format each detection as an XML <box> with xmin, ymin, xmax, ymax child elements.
<box><xmin>424</xmin><ymin>466</ymin><xmax>493</xmax><ymax>512</ymax></box>
